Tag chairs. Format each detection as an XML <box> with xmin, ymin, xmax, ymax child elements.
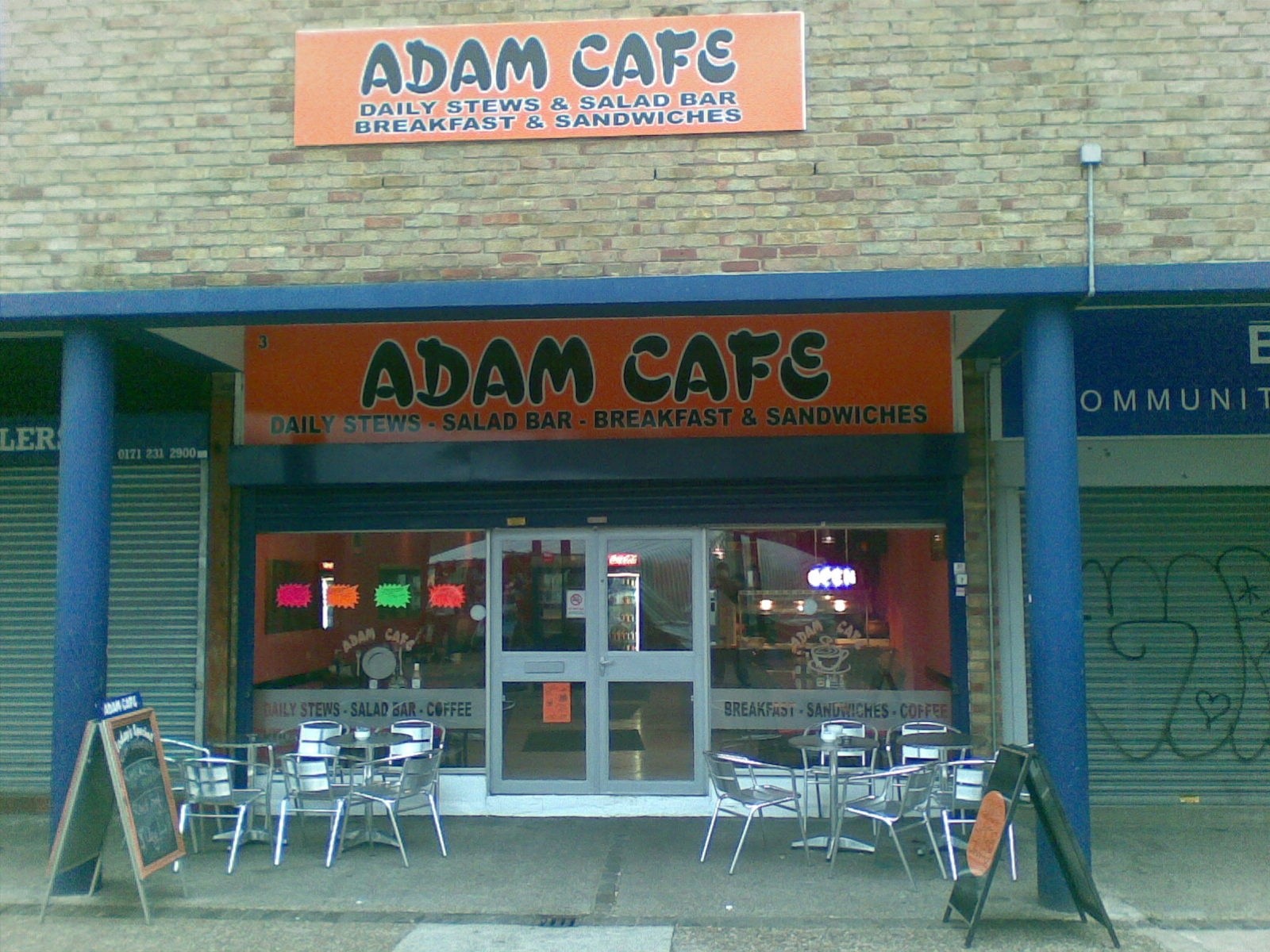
<box><xmin>158</xmin><ymin>737</ymin><xmax>225</xmax><ymax>854</ymax></box>
<box><xmin>884</xmin><ymin>718</ymin><xmax>968</xmax><ymax>765</ymax></box>
<box><xmin>699</xmin><ymin>746</ymin><xmax>813</xmax><ymax>875</ymax></box>
<box><xmin>801</xmin><ymin>718</ymin><xmax>883</xmax><ymax>837</ymax></box>
<box><xmin>173</xmin><ymin>757</ymin><xmax>271</xmax><ymax>875</ymax></box>
<box><xmin>277</xmin><ymin>719</ymin><xmax>351</xmax><ymax>830</ymax></box>
<box><xmin>933</xmin><ymin>759</ymin><xmax>1018</xmax><ymax>884</ymax></box>
<box><xmin>825</xmin><ymin>760</ymin><xmax>948</xmax><ymax>892</ymax></box>
<box><xmin>373</xmin><ymin>718</ymin><xmax>447</xmax><ymax>821</ymax></box>
<box><xmin>272</xmin><ymin>752</ymin><xmax>357</xmax><ymax>869</ymax></box>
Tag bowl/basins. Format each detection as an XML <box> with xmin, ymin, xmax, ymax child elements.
<box><xmin>354</xmin><ymin>731</ymin><xmax>371</xmax><ymax>740</ymax></box>
<box><xmin>821</xmin><ymin>733</ymin><xmax>836</xmax><ymax>743</ymax></box>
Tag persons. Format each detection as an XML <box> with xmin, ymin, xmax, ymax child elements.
<box><xmin>714</xmin><ymin>562</ymin><xmax>744</xmax><ymax>648</ymax></box>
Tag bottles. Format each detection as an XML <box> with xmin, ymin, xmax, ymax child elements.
<box><xmin>411</xmin><ymin>663</ymin><xmax>421</xmax><ymax>689</ymax></box>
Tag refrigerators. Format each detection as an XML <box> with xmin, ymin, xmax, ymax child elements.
<box><xmin>608</xmin><ymin>553</ymin><xmax>641</xmax><ymax>652</ymax></box>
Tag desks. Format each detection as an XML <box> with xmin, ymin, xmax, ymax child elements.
<box><xmin>894</xmin><ymin>732</ymin><xmax>988</xmax><ymax>856</ymax></box>
<box><xmin>785</xmin><ymin>733</ymin><xmax>881</xmax><ymax>855</ymax></box>
<box><xmin>205</xmin><ymin>731</ymin><xmax>299</xmax><ymax>853</ymax></box>
<box><xmin>323</xmin><ymin>731</ymin><xmax>413</xmax><ymax>852</ymax></box>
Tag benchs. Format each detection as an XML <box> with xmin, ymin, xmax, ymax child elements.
<box><xmin>338</xmin><ymin>746</ymin><xmax>449</xmax><ymax>868</ymax></box>
